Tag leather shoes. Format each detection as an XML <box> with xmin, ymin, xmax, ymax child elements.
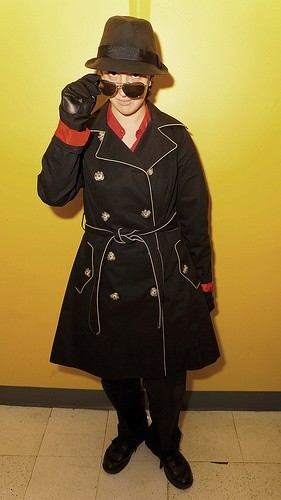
<box><xmin>102</xmin><ymin>435</ymin><xmax>138</xmax><ymax>474</ymax></box>
<box><xmin>159</xmin><ymin>450</ymin><xmax>193</xmax><ymax>489</ymax></box>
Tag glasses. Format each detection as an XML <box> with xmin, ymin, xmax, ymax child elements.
<box><xmin>96</xmin><ymin>78</ymin><xmax>152</xmax><ymax>100</ymax></box>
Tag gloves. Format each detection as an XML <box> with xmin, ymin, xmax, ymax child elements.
<box><xmin>60</xmin><ymin>73</ymin><xmax>102</xmax><ymax>123</ymax></box>
<box><xmin>204</xmin><ymin>292</ymin><xmax>215</xmax><ymax>313</ymax></box>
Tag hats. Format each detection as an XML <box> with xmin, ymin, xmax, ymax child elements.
<box><xmin>85</xmin><ymin>15</ymin><xmax>168</xmax><ymax>76</ymax></box>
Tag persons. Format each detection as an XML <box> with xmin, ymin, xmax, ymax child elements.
<box><xmin>36</xmin><ymin>15</ymin><xmax>220</xmax><ymax>489</ymax></box>
